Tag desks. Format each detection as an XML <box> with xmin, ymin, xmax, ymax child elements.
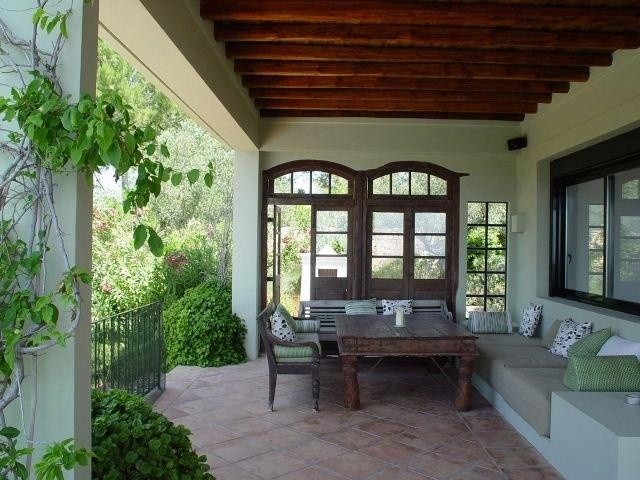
<box><xmin>335</xmin><ymin>314</ymin><xmax>478</xmax><ymax>411</ymax></box>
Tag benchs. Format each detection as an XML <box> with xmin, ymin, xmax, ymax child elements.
<box><xmin>257</xmin><ymin>298</ymin><xmax>321</xmax><ymax>412</ymax></box>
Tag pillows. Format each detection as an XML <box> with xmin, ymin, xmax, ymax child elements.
<box><xmin>597</xmin><ymin>335</ymin><xmax>640</xmax><ymax>363</ymax></box>
<box><xmin>344</xmin><ymin>298</ymin><xmax>377</xmax><ymax>315</ymax></box>
<box><xmin>272</xmin><ymin>313</ymin><xmax>296</xmax><ymax>342</ymax></box>
<box><xmin>566</xmin><ymin>327</ymin><xmax>611</xmax><ymax>358</ymax></box>
<box><xmin>277</xmin><ymin>303</ymin><xmax>297</xmax><ymax>332</ymax></box>
<box><xmin>468</xmin><ymin>310</ymin><xmax>512</xmax><ymax>333</ymax></box>
<box><xmin>519</xmin><ymin>303</ymin><xmax>543</xmax><ymax>339</ymax></box>
<box><xmin>382</xmin><ymin>299</ymin><xmax>412</xmax><ymax>315</ymax></box>
<box><xmin>564</xmin><ymin>355</ymin><xmax>640</xmax><ymax>392</ymax></box>
<box><xmin>541</xmin><ymin>319</ymin><xmax>561</xmax><ymax>348</ymax></box>
<box><xmin>548</xmin><ymin>319</ymin><xmax>592</xmax><ymax>358</ymax></box>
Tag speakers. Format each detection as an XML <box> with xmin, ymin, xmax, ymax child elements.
<box><xmin>507</xmin><ymin>137</ymin><xmax>527</xmax><ymax>150</ymax></box>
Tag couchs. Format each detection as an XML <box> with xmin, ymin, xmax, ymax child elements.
<box><xmin>299</xmin><ymin>300</ymin><xmax>454</xmax><ymax>356</ymax></box>
<box><xmin>460</xmin><ymin>322</ymin><xmax>640</xmax><ymax>479</ymax></box>
